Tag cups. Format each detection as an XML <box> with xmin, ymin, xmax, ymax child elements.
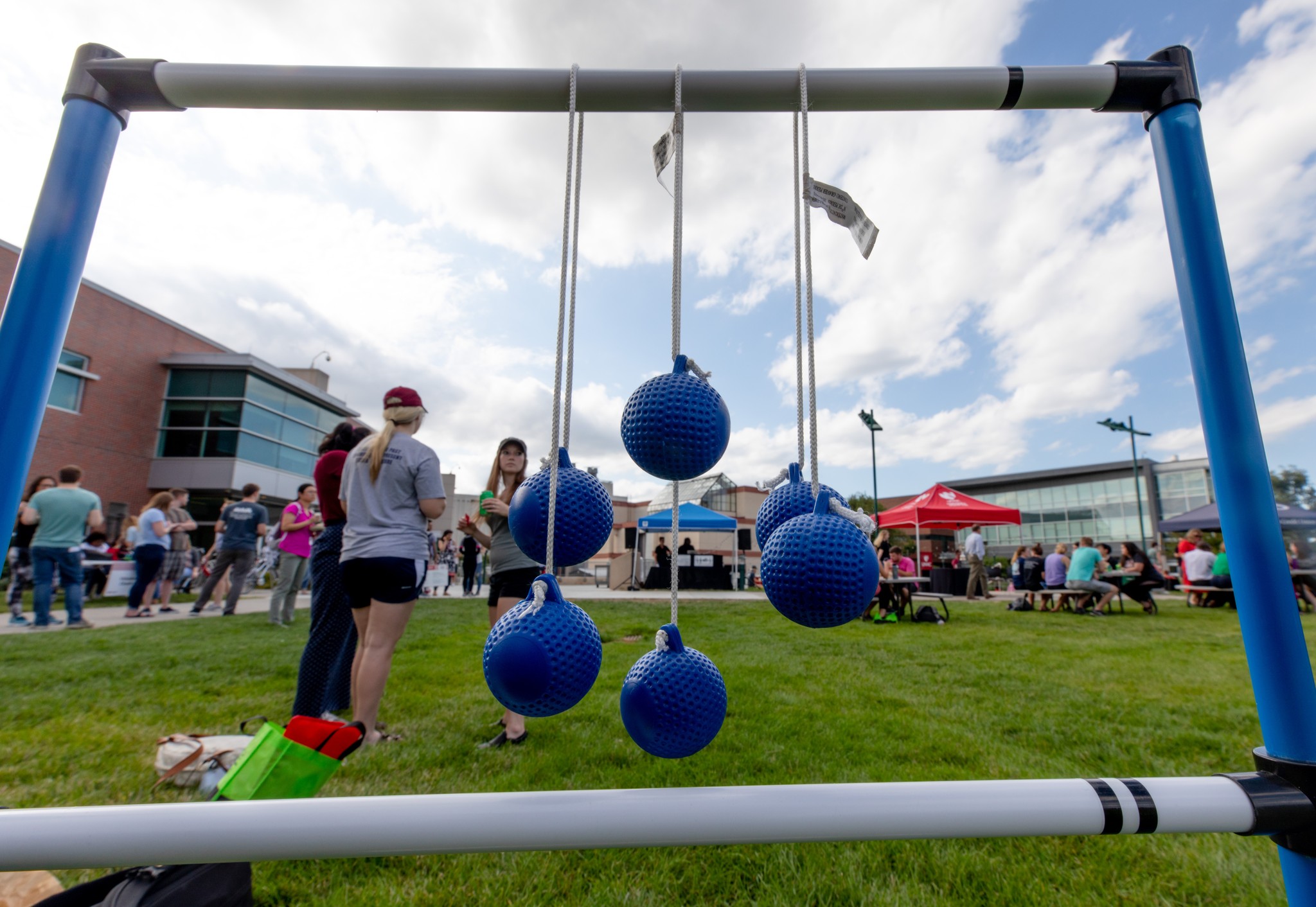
<box><xmin>1116</xmin><ymin>564</ymin><xmax>1121</xmax><ymax>570</ymax></box>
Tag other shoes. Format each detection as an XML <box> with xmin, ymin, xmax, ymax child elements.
<box><xmin>1074</xmin><ymin>607</ymin><xmax>1090</xmax><ymax>615</ymax></box>
<box><xmin>67</xmin><ymin>618</ymin><xmax>94</xmax><ymax>628</ymax></box>
<box><xmin>1090</xmin><ymin>609</ymin><xmax>1107</xmax><ymax>617</ymax></box>
<box><xmin>861</xmin><ymin>613</ymin><xmax>896</xmax><ymax>624</ymax></box>
<box><xmin>374</xmin><ymin>722</ymin><xmax>406</xmax><ymax>746</ymax></box>
<box><xmin>895</xmin><ymin>607</ymin><xmax>905</xmax><ymax>618</ymax></box>
<box><xmin>320</xmin><ymin>710</ymin><xmax>350</xmax><ymax>726</ymax></box>
<box><xmin>476</xmin><ymin>729</ymin><xmax>529</xmax><ymax>751</ymax></box>
<box><xmin>8</xmin><ymin>615</ymin><xmax>31</xmax><ymax>627</ymax></box>
<box><xmin>34</xmin><ymin>614</ymin><xmax>64</xmax><ymax>626</ymax></box>
<box><xmin>1143</xmin><ymin>602</ymin><xmax>1153</xmax><ymax>615</ymax></box>
<box><xmin>487</xmin><ymin>718</ymin><xmax>507</xmax><ymax>729</ymax></box>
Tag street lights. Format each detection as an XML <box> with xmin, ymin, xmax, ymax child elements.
<box><xmin>858</xmin><ymin>409</ymin><xmax>884</xmax><ymax>538</ymax></box>
<box><xmin>1096</xmin><ymin>414</ymin><xmax>1153</xmax><ymax>557</ymax></box>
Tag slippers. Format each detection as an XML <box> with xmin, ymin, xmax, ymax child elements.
<box><xmin>123</xmin><ymin>611</ymin><xmax>155</xmax><ymax>618</ymax></box>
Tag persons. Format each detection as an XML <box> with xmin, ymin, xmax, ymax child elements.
<box><xmin>333</xmin><ymin>387</ymin><xmax>447</xmax><ymax>745</ymax></box>
<box><xmin>1173</xmin><ymin>527</ymin><xmax>1236</xmax><ymax>609</ymax></box>
<box><xmin>678</xmin><ymin>538</ymin><xmax>694</xmax><ymax>554</ymax></box>
<box><xmin>124</xmin><ymin>484</ymin><xmax>268</xmax><ymax>617</ymax></box>
<box><xmin>81</xmin><ymin>515</ymin><xmax>163</xmax><ymax>606</ymax></box>
<box><xmin>431</xmin><ymin>529</ymin><xmax>458</xmax><ymax>596</ymax></box>
<box><xmin>654</xmin><ymin>537</ymin><xmax>671</xmax><ymax>568</ymax></box>
<box><xmin>952</xmin><ymin>549</ymin><xmax>961</xmax><ymax>568</ymax></box>
<box><xmin>458</xmin><ymin>437</ymin><xmax>546</xmax><ymax>749</ymax></box>
<box><xmin>291</xmin><ymin>421</ymin><xmax>372</xmax><ymax>723</ymax></box>
<box><xmin>6</xmin><ymin>474</ymin><xmax>57</xmax><ymax>628</ymax></box>
<box><xmin>858</xmin><ymin>529</ymin><xmax>919</xmax><ymax>621</ymax></box>
<box><xmin>1148</xmin><ymin>542</ymin><xmax>1162</xmax><ymax>568</ymax></box>
<box><xmin>265</xmin><ymin>483</ymin><xmax>320</xmax><ymax>629</ymax></box>
<box><xmin>964</xmin><ymin>524</ymin><xmax>996</xmax><ymax>601</ymax></box>
<box><xmin>1011</xmin><ymin>537</ymin><xmax>1122</xmax><ymax>617</ymax></box>
<box><xmin>1118</xmin><ymin>542</ymin><xmax>1164</xmax><ymax>615</ymax></box>
<box><xmin>459</xmin><ymin>532</ymin><xmax>484</xmax><ymax>597</ymax></box>
<box><xmin>1285</xmin><ymin>542</ymin><xmax>1316</xmax><ymax>614</ymax></box>
<box><xmin>20</xmin><ymin>464</ymin><xmax>106</xmax><ymax>630</ymax></box>
<box><xmin>419</xmin><ymin>520</ymin><xmax>439</xmax><ymax>594</ymax></box>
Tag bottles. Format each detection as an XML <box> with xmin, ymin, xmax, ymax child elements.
<box><xmin>892</xmin><ymin>564</ymin><xmax>899</xmax><ymax>579</ymax></box>
<box><xmin>887</xmin><ymin>567</ymin><xmax>893</xmax><ymax>580</ymax></box>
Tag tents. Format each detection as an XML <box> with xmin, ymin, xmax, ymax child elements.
<box><xmin>867</xmin><ymin>482</ymin><xmax>1023</xmax><ymax>594</ymax></box>
<box><xmin>1157</xmin><ymin>494</ymin><xmax>1316</xmax><ymax>532</ymax></box>
<box><xmin>631</xmin><ymin>501</ymin><xmax>738</xmax><ymax>592</ymax></box>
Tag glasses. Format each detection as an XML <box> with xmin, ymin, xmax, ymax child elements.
<box><xmin>1196</xmin><ymin>535</ymin><xmax>1201</xmax><ymax>538</ymax></box>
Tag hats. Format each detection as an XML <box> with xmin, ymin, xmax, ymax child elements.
<box><xmin>383</xmin><ymin>386</ymin><xmax>428</xmax><ymax>413</ymax></box>
<box><xmin>498</xmin><ymin>437</ymin><xmax>527</xmax><ymax>457</ymax></box>
<box><xmin>752</xmin><ymin>565</ymin><xmax>757</xmax><ymax>569</ymax></box>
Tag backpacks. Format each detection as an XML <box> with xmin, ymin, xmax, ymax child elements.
<box><xmin>1007</xmin><ymin>597</ymin><xmax>1033</xmax><ymax>611</ymax></box>
<box><xmin>264</xmin><ymin>501</ymin><xmax>315</xmax><ymax>552</ymax></box>
<box><xmin>913</xmin><ymin>604</ymin><xmax>946</xmax><ymax>623</ymax></box>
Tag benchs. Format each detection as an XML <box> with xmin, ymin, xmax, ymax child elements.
<box><xmin>1175</xmin><ymin>585</ymin><xmax>1233</xmax><ymax>608</ymax></box>
<box><xmin>1008</xmin><ymin>589</ymin><xmax>1091</xmax><ymax>611</ymax></box>
<box><xmin>898</xmin><ymin>592</ymin><xmax>953</xmax><ymax>621</ymax></box>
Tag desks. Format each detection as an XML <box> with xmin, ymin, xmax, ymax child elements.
<box><xmin>1098</xmin><ymin>570</ymin><xmax>1142</xmax><ymax>613</ymax></box>
<box><xmin>879</xmin><ymin>577</ymin><xmax>931</xmax><ymax>619</ymax></box>
<box><xmin>644</xmin><ymin>566</ymin><xmax>734</xmax><ymax>590</ymax></box>
<box><xmin>1290</xmin><ymin>569</ymin><xmax>1316</xmax><ymax>613</ymax></box>
<box><xmin>921</xmin><ymin>568</ymin><xmax>984</xmax><ymax>596</ymax></box>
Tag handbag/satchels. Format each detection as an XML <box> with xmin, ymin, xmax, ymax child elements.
<box><xmin>24</xmin><ymin>862</ymin><xmax>257</xmax><ymax>907</ymax></box>
<box><xmin>147</xmin><ymin>716</ymin><xmax>269</xmax><ymax>794</ymax></box>
<box><xmin>175</xmin><ymin>750</ymin><xmax>244</xmax><ymax>801</ymax></box>
<box><xmin>423</xmin><ymin>563</ymin><xmax>449</xmax><ymax>587</ymax></box>
<box><xmin>202</xmin><ymin>714</ymin><xmax>367</xmax><ymax>800</ymax></box>
